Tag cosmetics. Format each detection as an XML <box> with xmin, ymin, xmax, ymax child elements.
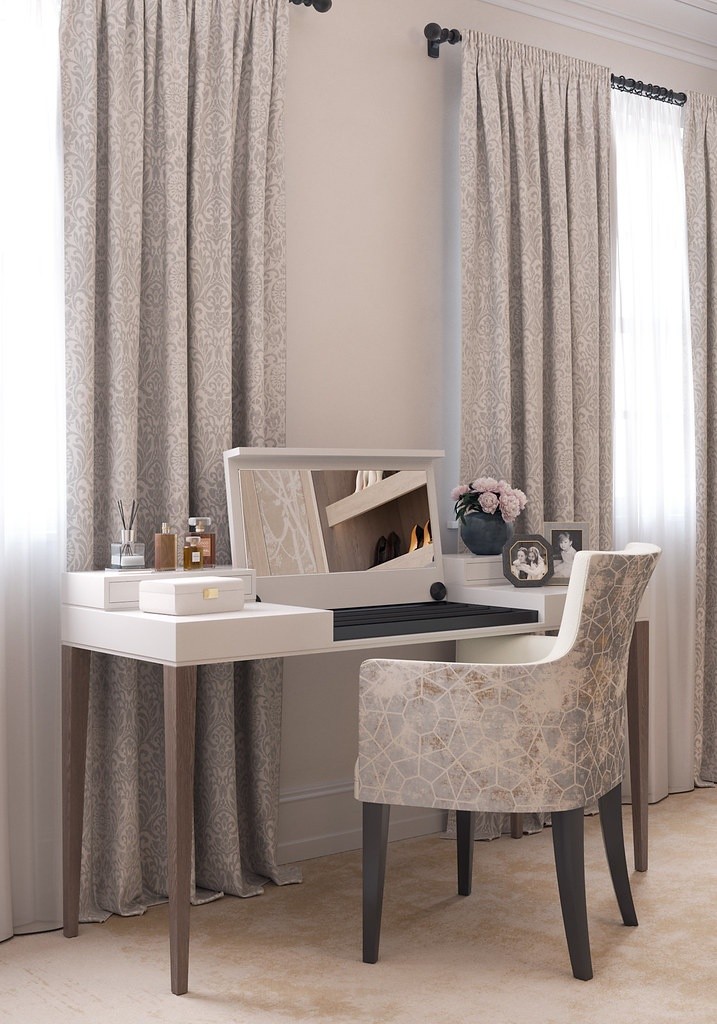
<box><xmin>155</xmin><ymin>523</ymin><xmax>178</xmax><ymax>572</ymax></box>
<box><xmin>189</xmin><ymin>517</ymin><xmax>217</xmax><ymax>569</ymax></box>
<box><xmin>184</xmin><ymin>537</ymin><xmax>204</xmax><ymax>571</ymax></box>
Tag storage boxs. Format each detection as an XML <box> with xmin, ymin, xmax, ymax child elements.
<box><xmin>63</xmin><ymin>566</ymin><xmax>333</xmax><ymax>664</ymax></box>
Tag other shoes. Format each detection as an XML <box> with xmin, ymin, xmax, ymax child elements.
<box><xmin>409</xmin><ymin>524</ymin><xmax>423</xmax><ymax>552</ymax></box>
<box><xmin>375</xmin><ymin>536</ymin><xmax>387</xmax><ymax>569</ymax></box>
<box><xmin>422</xmin><ymin>520</ymin><xmax>432</xmax><ymax>546</ymax></box>
<box><xmin>387</xmin><ymin>531</ymin><xmax>402</xmax><ymax>561</ymax></box>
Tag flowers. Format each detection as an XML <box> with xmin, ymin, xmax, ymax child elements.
<box><xmin>452</xmin><ymin>477</ymin><xmax>528</xmax><ymax>525</ymax></box>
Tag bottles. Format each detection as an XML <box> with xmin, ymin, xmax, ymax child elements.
<box><xmin>111</xmin><ymin>530</ymin><xmax>145</xmax><ymax>568</ymax></box>
<box><xmin>155</xmin><ymin>517</ymin><xmax>215</xmax><ymax>571</ymax></box>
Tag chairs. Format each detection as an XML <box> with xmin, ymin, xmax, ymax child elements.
<box><xmin>354</xmin><ymin>543</ymin><xmax>661</xmax><ymax>980</ymax></box>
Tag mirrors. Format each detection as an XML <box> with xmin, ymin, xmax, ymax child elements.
<box><xmin>223</xmin><ymin>447</ymin><xmax>446</xmax><ymax>609</ymax></box>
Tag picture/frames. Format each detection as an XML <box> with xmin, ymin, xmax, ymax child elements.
<box><xmin>502</xmin><ymin>534</ymin><xmax>554</xmax><ymax>588</ymax></box>
<box><xmin>543</xmin><ymin>522</ymin><xmax>588</xmax><ymax>586</ymax></box>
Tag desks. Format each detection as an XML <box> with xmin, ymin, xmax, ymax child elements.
<box><xmin>61</xmin><ymin>570</ymin><xmax>650</xmax><ymax>996</ymax></box>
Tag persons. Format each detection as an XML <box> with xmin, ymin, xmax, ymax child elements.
<box><xmin>554</xmin><ymin>532</ymin><xmax>577</xmax><ymax>578</ymax></box>
<box><xmin>511</xmin><ymin>546</ymin><xmax>545</xmax><ymax>580</ymax></box>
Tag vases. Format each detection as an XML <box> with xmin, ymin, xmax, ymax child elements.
<box><xmin>460</xmin><ymin>512</ymin><xmax>507</xmax><ymax>555</ymax></box>
